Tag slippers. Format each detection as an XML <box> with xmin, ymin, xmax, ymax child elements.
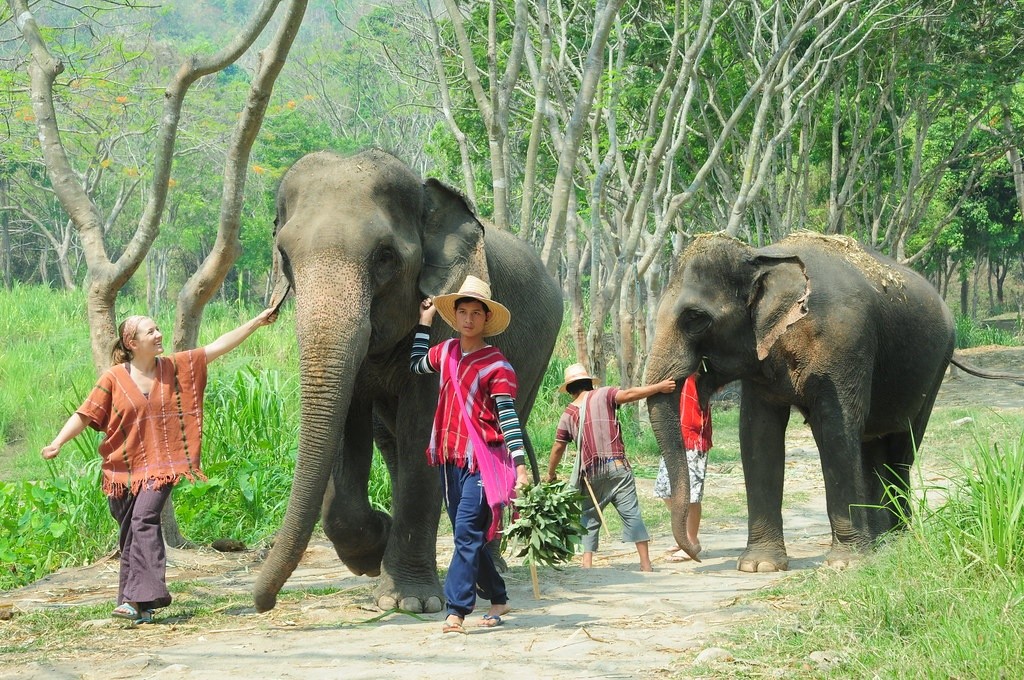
<box><xmin>443</xmin><ymin>621</ymin><xmax>463</xmax><ymax>632</ymax></box>
<box><xmin>667</xmin><ymin>546</ymin><xmax>681</xmax><ymax>551</ymax></box>
<box><xmin>667</xmin><ymin>553</ymin><xmax>692</xmax><ymax>563</ymax></box>
<box><xmin>476</xmin><ymin>614</ymin><xmax>505</xmax><ymax>627</ymax></box>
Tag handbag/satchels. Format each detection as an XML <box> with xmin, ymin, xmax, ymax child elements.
<box><xmin>568</xmin><ymin>450</ymin><xmax>581</xmax><ymax>491</ymax></box>
<box><xmin>464</xmin><ymin>420</ymin><xmax>519</xmax><ymax>542</ymax></box>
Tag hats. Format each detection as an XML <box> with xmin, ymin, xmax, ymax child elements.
<box><xmin>558</xmin><ymin>363</ymin><xmax>601</xmax><ymax>393</ymax></box>
<box><xmin>433</xmin><ymin>275</ymin><xmax>511</xmax><ymax>337</ymax></box>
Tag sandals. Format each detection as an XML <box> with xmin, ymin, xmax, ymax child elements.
<box><xmin>111</xmin><ymin>604</ymin><xmax>139</xmax><ymax>619</ymax></box>
<box><xmin>135</xmin><ymin>608</ymin><xmax>155</xmax><ymax>624</ymax></box>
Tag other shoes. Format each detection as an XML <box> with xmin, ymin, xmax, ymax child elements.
<box><xmin>652</xmin><ymin>568</ymin><xmax>661</xmax><ymax>572</ymax></box>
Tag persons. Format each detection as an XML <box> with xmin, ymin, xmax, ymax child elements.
<box><xmin>653</xmin><ymin>357</ymin><xmax>724</xmax><ymax>563</ymax></box>
<box><xmin>545</xmin><ymin>363</ymin><xmax>676</xmax><ymax>573</ymax></box>
<box><xmin>409</xmin><ymin>274</ymin><xmax>529</xmax><ymax>635</ymax></box>
<box><xmin>41</xmin><ymin>307</ymin><xmax>279</xmax><ymax>620</ymax></box>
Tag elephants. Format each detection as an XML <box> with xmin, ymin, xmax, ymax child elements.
<box><xmin>252</xmin><ymin>147</ymin><xmax>564</xmax><ymax>615</ymax></box>
<box><xmin>645</xmin><ymin>228</ymin><xmax>1024</xmax><ymax>572</ymax></box>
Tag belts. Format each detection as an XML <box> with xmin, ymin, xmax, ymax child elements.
<box><xmin>590</xmin><ymin>458</ymin><xmax>618</xmax><ymax>470</ymax></box>
